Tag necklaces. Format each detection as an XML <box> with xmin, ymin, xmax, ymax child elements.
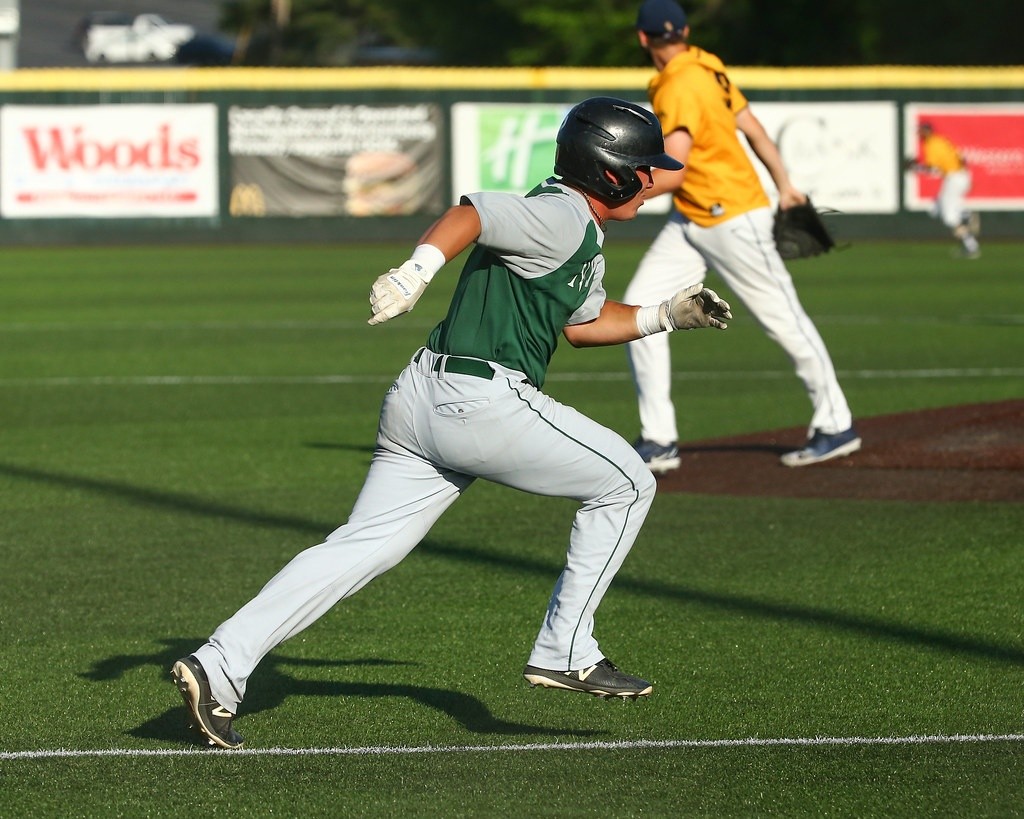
<box><xmin>571</xmin><ymin>186</ymin><xmax>608</xmax><ymax>233</ymax></box>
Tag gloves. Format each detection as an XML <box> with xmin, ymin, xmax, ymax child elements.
<box><xmin>367</xmin><ymin>260</ymin><xmax>433</xmax><ymax>326</ymax></box>
<box><xmin>658</xmin><ymin>282</ymin><xmax>733</xmax><ymax>333</ymax></box>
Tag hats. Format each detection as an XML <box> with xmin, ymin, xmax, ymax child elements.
<box><xmin>637</xmin><ymin>0</ymin><xmax>687</xmax><ymax>39</ymax></box>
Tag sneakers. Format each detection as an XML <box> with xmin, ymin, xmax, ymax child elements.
<box><xmin>170</xmin><ymin>655</ymin><xmax>245</xmax><ymax>749</ymax></box>
<box><xmin>522</xmin><ymin>658</ymin><xmax>653</xmax><ymax>699</ymax></box>
<box><xmin>781</xmin><ymin>427</ymin><xmax>862</xmax><ymax>467</ymax></box>
<box><xmin>632</xmin><ymin>435</ymin><xmax>682</xmax><ymax>474</ymax></box>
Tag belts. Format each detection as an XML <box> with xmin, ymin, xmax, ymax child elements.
<box><xmin>414</xmin><ymin>349</ymin><xmax>528</xmax><ymax>384</ymax></box>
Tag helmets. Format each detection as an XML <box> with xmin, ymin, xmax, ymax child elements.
<box><xmin>553</xmin><ymin>96</ymin><xmax>684</xmax><ymax>200</ymax></box>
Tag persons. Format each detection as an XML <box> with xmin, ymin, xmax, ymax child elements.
<box><xmin>622</xmin><ymin>0</ymin><xmax>861</xmax><ymax>472</ymax></box>
<box><xmin>904</xmin><ymin>117</ymin><xmax>981</xmax><ymax>262</ymax></box>
<box><xmin>170</xmin><ymin>99</ymin><xmax>733</xmax><ymax>748</ymax></box>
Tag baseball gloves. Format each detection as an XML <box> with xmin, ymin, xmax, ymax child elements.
<box><xmin>903</xmin><ymin>158</ymin><xmax>918</xmax><ymax>169</ymax></box>
<box><xmin>772</xmin><ymin>193</ymin><xmax>852</xmax><ymax>260</ymax></box>
